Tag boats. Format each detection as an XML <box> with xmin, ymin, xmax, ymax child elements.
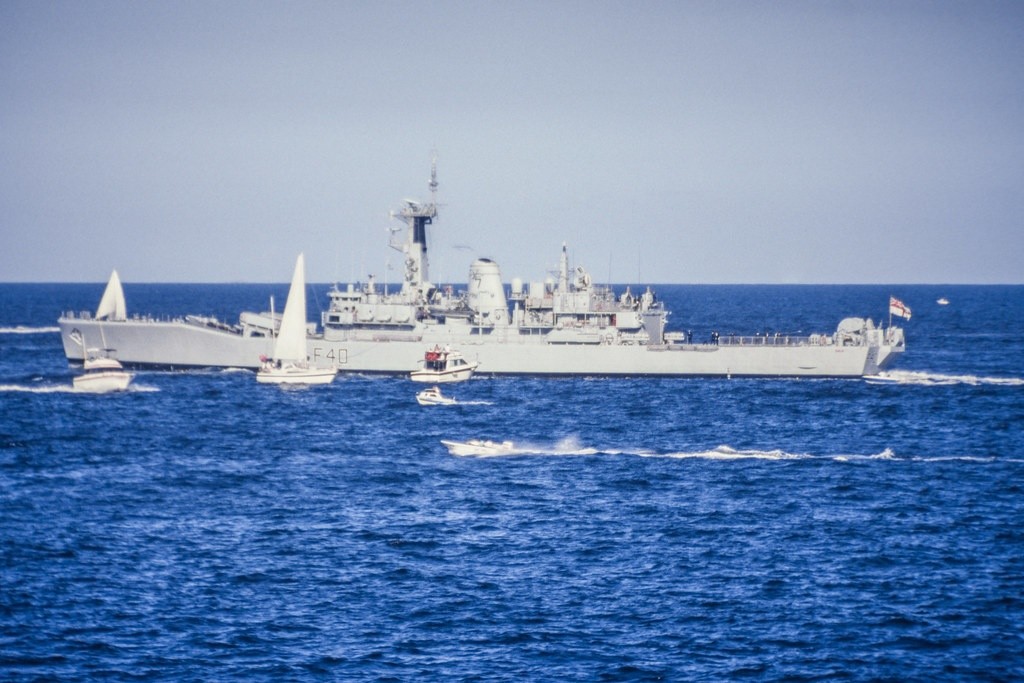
<box><xmin>55</xmin><ymin>157</ymin><xmax>916</xmax><ymax>380</ymax></box>
<box><xmin>255</xmin><ymin>355</ymin><xmax>338</xmax><ymax>387</ymax></box>
<box><xmin>440</xmin><ymin>437</ymin><xmax>514</xmax><ymax>460</ymax></box>
<box><xmin>415</xmin><ymin>385</ymin><xmax>455</xmax><ymax>409</ymax></box>
<box><xmin>72</xmin><ymin>318</ymin><xmax>133</xmax><ymax>394</ymax></box>
<box><xmin>409</xmin><ymin>341</ymin><xmax>477</xmax><ymax>383</ymax></box>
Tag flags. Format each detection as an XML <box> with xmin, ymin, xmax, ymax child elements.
<box><xmin>888</xmin><ymin>297</ymin><xmax>912</xmax><ymax>321</ymax></box>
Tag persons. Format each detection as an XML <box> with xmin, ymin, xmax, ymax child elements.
<box><xmin>612</xmin><ymin>287</ymin><xmax>659</xmax><ymax>312</ymax></box>
<box><xmin>710</xmin><ymin>329</ymin><xmax>782</xmax><ymax>346</ymax></box>
<box><xmin>687</xmin><ymin>329</ymin><xmax>692</xmax><ymax>344</ymax></box>
